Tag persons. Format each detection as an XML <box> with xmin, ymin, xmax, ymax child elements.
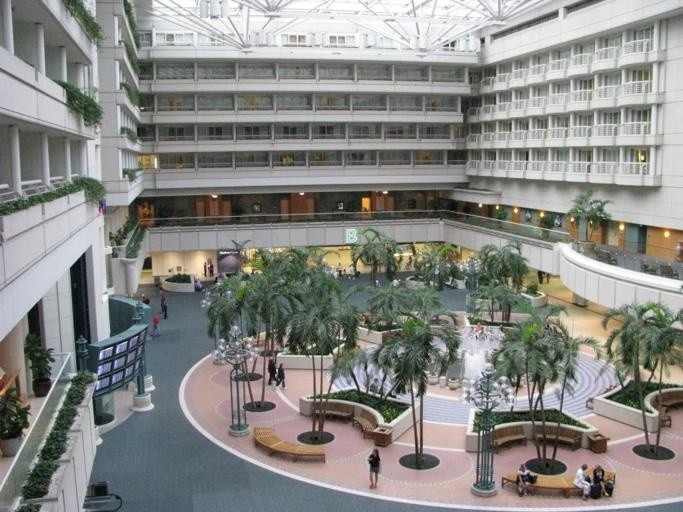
<box><xmin>365</xmin><ymin>448</ymin><xmax>382</xmax><ymax>490</ymax></box>
<box><xmin>266</xmin><ymin>358</ymin><xmax>276</xmax><ymax>385</ymax></box>
<box><xmin>337</xmin><ymin>262</ymin><xmax>343</xmax><ymax>279</ymax></box>
<box><xmin>514</xmin><ymin>462</ymin><xmax>615</xmax><ymax>501</ymax></box>
<box><xmin>208</xmin><ymin>262</ymin><xmax>214</xmax><ymax>278</ymax></box>
<box><xmin>151</xmin><ymin>312</ymin><xmax>161</xmax><ymax>336</ymax></box>
<box><xmin>204</xmin><ymin>262</ymin><xmax>207</xmax><ymax>277</ymax></box>
<box><xmin>537</xmin><ymin>271</ymin><xmax>550</xmax><ymax>284</ymax></box>
<box><xmin>348</xmin><ymin>263</ymin><xmax>357</xmax><ymax>280</ymax></box>
<box><xmin>143</xmin><ymin>294</ymin><xmax>150</xmax><ymax>304</ymax></box>
<box><xmin>275</xmin><ymin>363</ymin><xmax>287</xmax><ymax>389</ymax></box>
<box><xmin>160</xmin><ymin>291</ymin><xmax>169</xmax><ymax>320</ymax></box>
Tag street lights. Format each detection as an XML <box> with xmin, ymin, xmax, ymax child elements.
<box><xmin>463</xmin><ymin>363</ymin><xmax>512</xmax><ymax>497</ymax></box>
<box><xmin>200</xmin><ymin>280</ymin><xmax>236</xmax><ymax>363</ymax></box>
<box><xmin>212</xmin><ymin>324</ymin><xmax>260</xmax><ymax>436</ymax></box>
<box><xmin>322</xmin><ymin>257</ymin><xmax>340</xmax><ymax>280</ymax></box>
<box><xmin>461</xmin><ymin>253</ymin><xmax>483</xmax><ymax>313</ymax></box>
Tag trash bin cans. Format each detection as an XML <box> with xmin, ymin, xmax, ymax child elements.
<box><xmin>94</xmin><ymin>480</ymin><xmax>110</xmax><ymax>495</ymax></box>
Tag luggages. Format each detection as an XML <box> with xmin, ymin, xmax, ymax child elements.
<box><xmin>603</xmin><ymin>482</ymin><xmax>613</xmax><ymax>496</ymax></box>
<box><xmin>590</xmin><ymin>485</ymin><xmax>600</xmax><ymax>498</ymax></box>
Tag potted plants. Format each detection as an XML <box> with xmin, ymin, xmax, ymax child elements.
<box><xmin>560</xmin><ymin>184</ymin><xmax>617</xmax><ymax>254</ymax></box>
<box><xmin>22</xmin><ymin>333</ymin><xmax>55</xmax><ymax>397</ymax></box>
<box><xmin>0</xmin><ymin>387</ymin><xmax>32</xmax><ymax>457</ymax></box>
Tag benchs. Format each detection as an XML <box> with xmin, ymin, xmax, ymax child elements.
<box><xmin>501</xmin><ymin>469</ymin><xmax>615</xmax><ymax>498</ymax></box>
<box><xmin>483</xmin><ymin>422</ymin><xmax>528</xmax><ymax>454</ymax></box>
<box><xmin>306</xmin><ymin>398</ymin><xmax>356</xmax><ymax>426</ymax></box>
<box><xmin>530</xmin><ymin>421</ymin><xmax>585</xmax><ymax>454</ymax></box>
<box><xmin>253</xmin><ymin>425</ymin><xmax>326</xmax><ymax>463</ymax></box>
<box><xmin>657</xmin><ymin>386</ymin><xmax>681</xmax><ymax>409</ymax></box>
<box><xmin>351</xmin><ymin>406</ymin><xmax>379</xmax><ymax>439</ymax></box>
<box><xmin>649</xmin><ymin>389</ymin><xmax>671</xmax><ymax>427</ymax></box>
